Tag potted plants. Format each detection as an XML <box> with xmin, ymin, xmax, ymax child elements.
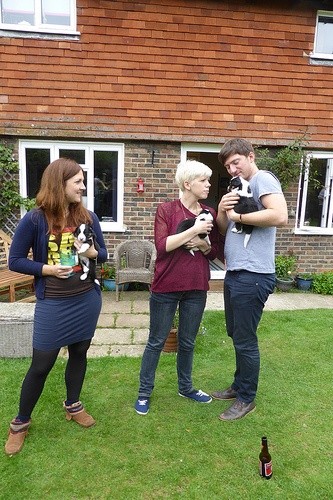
<box><xmin>274</xmin><ymin>253</ymin><xmax>298</xmax><ymax>292</ymax></box>
<box><xmin>162</xmin><ymin>308</ymin><xmax>179</xmax><ymax>353</ymax></box>
<box><xmin>296</xmin><ymin>273</ymin><xmax>317</xmax><ymax>291</ymax></box>
<box><xmin>99</xmin><ymin>254</ymin><xmax>130</xmax><ymax>291</ymax></box>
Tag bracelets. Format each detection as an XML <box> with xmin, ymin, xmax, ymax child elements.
<box><xmin>240</xmin><ymin>214</ymin><xmax>241</xmax><ymax>222</ymax></box>
<box><xmin>203</xmin><ymin>248</ymin><xmax>210</xmax><ymax>253</ymax></box>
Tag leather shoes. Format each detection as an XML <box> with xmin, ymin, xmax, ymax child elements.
<box><xmin>210</xmin><ymin>386</ymin><xmax>239</xmax><ymax>400</ymax></box>
<box><xmin>218</xmin><ymin>398</ymin><xmax>256</xmax><ymax>421</ymax></box>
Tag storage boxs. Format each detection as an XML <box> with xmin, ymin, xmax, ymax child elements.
<box><xmin>0</xmin><ymin>302</ymin><xmax>65</xmax><ymax>357</ymax></box>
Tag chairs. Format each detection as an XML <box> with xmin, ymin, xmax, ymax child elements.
<box><xmin>114</xmin><ymin>239</ymin><xmax>158</xmax><ymax>302</ymax></box>
<box><xmin>0</xmin><ymin>228</ymin><xmax>37</xmax><ymax>303</ymax></box>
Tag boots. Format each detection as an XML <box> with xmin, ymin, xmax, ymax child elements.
<box><xmin>63</xmin><ymin>400</ymin><xmax>95</xmax><ymax>427</ymax></box>
<box><xmin>4</xmin><ymin>419</ymin><xmax>31</xmax><ymax>454</ymax></box>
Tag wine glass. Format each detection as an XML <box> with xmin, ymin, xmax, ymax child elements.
<box><xmin>59</xmin><ymin>250</ymin><xmax>76</xmax><ymax>277</ymax></box>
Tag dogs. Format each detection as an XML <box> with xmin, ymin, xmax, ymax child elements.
<box><xmin>227</xmin><ymin>174</ymin><xmax>259</xmax><ymax>248</ymax></box>
<box><xmin>177</xmin><ymin>209</ymin><xmax>214</xmax><ymax>257</ymax></box>
<box><xmin>73</xmin><ymin>223</ymin><xmax>100</xmax><ymax>285</ymax></box>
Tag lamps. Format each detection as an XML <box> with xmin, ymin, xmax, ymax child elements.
<box><xmin>137</xmin><ymin>177</ymin><xmax>145</xmax><ymax>194</ymax></box>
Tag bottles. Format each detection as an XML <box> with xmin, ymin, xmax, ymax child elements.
<box><xmin>259</xmin><ymin>436</ymin><xmax>273</xmax><ymax>480</ymax></box>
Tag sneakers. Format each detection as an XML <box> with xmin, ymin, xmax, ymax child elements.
<box><xmin>178</xmin><ymin>387</ymin><xmax>212</xmax><ymax>403</ymax></box>
<box><xmin>134</xmin><ymin>396</ymin><xmax>149</xmax><ymax>415</ymax></box>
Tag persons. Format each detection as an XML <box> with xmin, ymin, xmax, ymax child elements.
<box><xmin>134</xmin><ymin>159</ymin><xmax>217</xmax><ymax>416</ymax></box>
<box><xmin>318</xmin><ymin>188</ymin><xmax>324</xmax><ymax>227</ymax></box>
<box><xmin>4</xmin><ymin>158</ymin><xmax>107</xmax><ymax>455</ymax></box>
<box><xmin>94</xmin><ymin>174</ymin><xmax>107</xmax><ymax>221</ymax></box>
<box><xmin>210</xmin><ymin>139</ymin><xmax>288</xmax><ymax>422</ymax></box>
